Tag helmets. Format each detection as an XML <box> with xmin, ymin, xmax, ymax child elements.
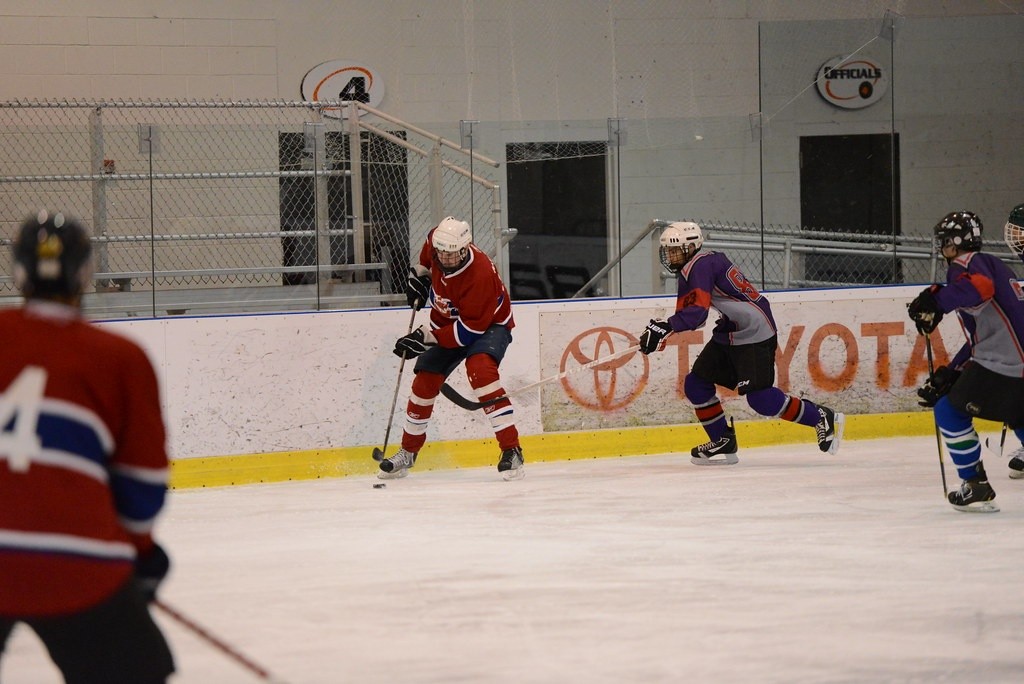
<box><xmin>935</xmin><ymin>212</ymin><xmax>983</xmax><ymax>250</ymax></box>
<box><xmin>658</xmin><ymin>221</ymin><xmax>704</xmax><ymax>274</ymax></box>
<box><xmin>1003</xmin><ymin>205</ymin><xmax>1024</xmax><ymax>261</ymax></box>
<box><xmin>14</xmin><ymin>211</ymin><xmax>91</xmax><ymax>299</ymax></box>
<box><xmin>432</xmin><ymin>216</ymin><xmax>472</xmax><ymax>273</ymax></box>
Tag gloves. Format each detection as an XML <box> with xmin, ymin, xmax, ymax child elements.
<box><xmin>136</xmin><ymin>540</ymin><xmax>169</xmax><ymax>603</ymax></box>
<box><xmin>640</xmin><ymin>318</ymin><xmax>674</xmax><ymax>356</ymax></box>
<box><xmin>392</xmin><ymin>328</ymin><xmax>430</xmax><ymax>360</ymax></box>
<box><xmin>908</xmin><ymin>283</ymin><xmax>948</xmax><ymax>335</ymax></box>
<box><xmin>406</xmin><ymin>264</ymin><xmax>430</xmax><ymax>310</ymax></box>
<box><xmin>917</xmin><ymin>365</ymin><xmax>961</xmax><ymax>407</ymax></box>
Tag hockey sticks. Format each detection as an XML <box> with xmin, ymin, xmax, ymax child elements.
<box><xmin>984</xmin><ymin>421</ymin><xmax>1009</xmax><ymax>457</ymax></box>
<box><xmin>371</xmin><ymin>298</ymin><xmax>419</xmax><ymax>461</ymax></box>
<box><xmin>922</xmin><ymin>307</ymin><xmax>949</xmax><ymax>501</ymax></box>
<box><xmin>151</xmin><ymin>598</ymin><xmax>282</xmax><ymax>683</ymax></box>
<box><xmin>438</xmin><ymin>343</ymin><xmax>641</xmax><ymax>411</ymax></box>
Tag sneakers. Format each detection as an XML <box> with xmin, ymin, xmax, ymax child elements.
<box><xmin>948</xmin><ymin>471</ymin><xmax>1000</xmax><ymax>513</ymax></box>
<box><xmin>691</xmin><ymin>428</ymin><xmax>738</xmax><ymax>464</ymax></box>
<box><xmin>377</xmin><ymin>450</ymin><xmax>418</xmax><ymax>479</ymax></box>
<box><xmin>497</xmin><ymin>445</ymin><xmax>525</xmax><ymax>480</ymax></box>
<box><xmin>804</xmin><ymin>398</ymin><xmax>844</xmax><ymax>455</ymax></box>
<box><xmin>1008</xmin><ymin>451</ymin><xmax>1024</xmax><ymax>479</ymax></box>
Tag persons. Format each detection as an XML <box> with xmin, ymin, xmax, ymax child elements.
<box><xmin>0</xmin><ymin>212</ymin><xmax>175</xmax><ymax>684</ymax></box>
<box><xmin>638</xmin><ymin>222</ymin><xmax>835</xmax><ymax>459</ymax></box>
<box><xmin>908</xmin><ymin>203</ymin><xmax>1024</xmax><ymax>506</ymax></box>
<box><xmin>379</xmin><ymin>216</ymin><xmax>524</xmax><ymax>473</ymax></box>
